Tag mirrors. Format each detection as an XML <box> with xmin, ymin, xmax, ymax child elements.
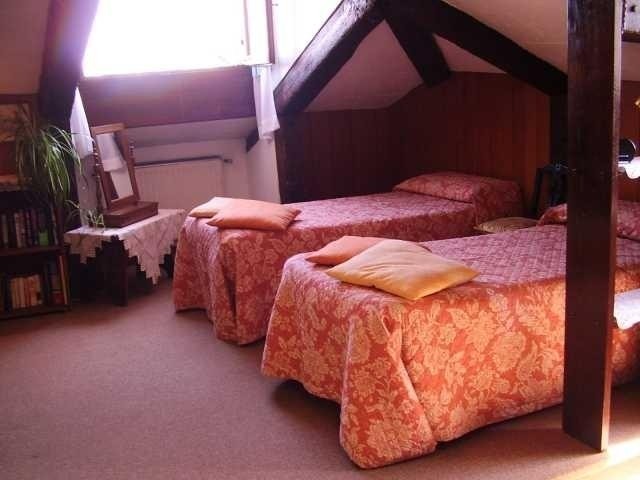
<box><xmin>90</xmin><ymin>121</ymin><xmax>142</xmax><ymax>212</ymax></box>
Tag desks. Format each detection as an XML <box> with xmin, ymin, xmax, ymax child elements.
<box><xmin>68</xmin><ymin>209</ymin><xmax>184</xmax><ymax>307</ymax></box>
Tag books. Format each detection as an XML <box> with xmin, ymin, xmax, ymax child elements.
<box><xmin>1</xmin><ymin>207</ymin><xmax>53</xmax><ymax>249</ymax></box>
<box><xmin>6</xmin><ymin>256</ymin><xmax>67</xmax><ymax>306</ymax></box>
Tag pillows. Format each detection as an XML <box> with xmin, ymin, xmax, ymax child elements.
<box><xmin>392</xmin><ymin>170</ymin><xmax>532</xmax><ymax>213</ymax></box>
<box><xmin>206</xmin><ymin>198</ymin><xmax>301</xmax><ymax>236</ymax></box>
<box><xmin>473</xmin><ymin>216</ymin><xmax>538</xmax><ymax>235</ymax></box>
<box><xmin>323</xmin><ymin>238</ymin><xmax>480</xmax><ymax>301</ymax></box>
<box><xmin>188</xmin><ymin>195</ymin><xmax>240</xmax><ymax>219</ymax></box>
<box><xmin>536</xmin><ymin>199</ymin><xmax>640</xmax><ymax>241</ymax></box>
<box><xmin>305</xmin><ymin>235</ymin><xmax>434</xmax><ymax>267</ymax></box>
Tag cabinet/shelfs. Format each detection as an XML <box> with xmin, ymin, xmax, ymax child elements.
<box><xmin>0</xmin><ymin>93</ymin><xmax>72</xmax><ymax>319</ymax></box>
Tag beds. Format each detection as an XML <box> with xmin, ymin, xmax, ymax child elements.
<box><xmin>259</xmin><ymin>221</ymin><xmax>640</xmax><ymax>470</ymax></box>
<box><xmin>173</xmin><ymin>170</ymin><xmax>523</xmax><ymax>345</ymax></box>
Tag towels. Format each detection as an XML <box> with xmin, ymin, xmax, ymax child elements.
<box><xmin>251</xmin><ymin>62</ymin><xmax>281</xmax><ymax>144</ymax></box>
<box><xmin>97</xmin><ymin>132</ymin><xmax>134</xmax><ymax>199</ymax></box>
<box><xmin>70</xmin><ymin>86</ymin><xmax>94</xmax><ymax>160</ymax></box>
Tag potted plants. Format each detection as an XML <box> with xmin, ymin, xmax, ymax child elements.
<box><xmin>12</xmin><ymin>121</ymin><xmax>105</xmax><ymax>226</ymax></box>
